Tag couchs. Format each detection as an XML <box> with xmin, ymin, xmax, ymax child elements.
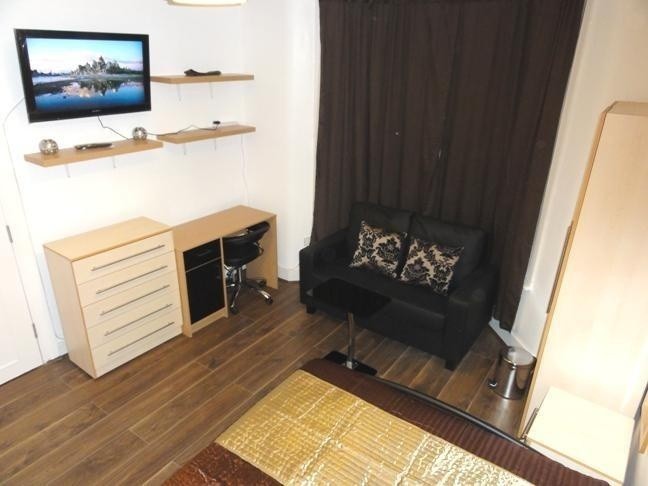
<box><xmin>296</xmin><ymin>202</ymin><xmax>499</xmax><ymax>372</ymax></box>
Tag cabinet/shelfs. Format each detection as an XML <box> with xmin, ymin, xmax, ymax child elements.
<box><xmin>516</xmin><ymin>96</ymin><xmax>648</xmax><ymax>485</ymax></box>
<box><xmin>40</xmin><ymin>215</ymin><xmax>184</xmax><ymax>379</ymax></box>
<box><xmin>152</xmin><ymin>71</ymin><xmax>256</xmax><ymax>146</ymax></box>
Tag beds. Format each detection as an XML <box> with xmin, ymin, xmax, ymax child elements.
<box><xmin>164</xmin><ymin>353</ymin><xmax>620</xmax><ymax>485</ymax></box>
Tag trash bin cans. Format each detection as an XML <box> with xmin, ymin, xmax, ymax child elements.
<box><xmin>488</xmin><ymin>346</ymin><xmax>537</xmax><ymax>400</ymax></box>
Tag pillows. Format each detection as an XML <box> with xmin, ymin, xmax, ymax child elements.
<box><xmin>398</xmin><ymin>234</ymin><xmax>467</xmax><ymax>299</ymax></box>
<box><xmin>350</xmin><ymin>218</ymin><xmax>407</xmax><ymax>278</ymax></box>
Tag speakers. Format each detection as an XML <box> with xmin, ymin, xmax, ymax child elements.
<box><xmin>133</xmin><ymin>127</ymin><xmax>145</xmax><ymax>140</ymax></box>
<box><xmin>39</xmin><ymin>138</ymin><xmax>58</xmax><ymax>155</ymax></box>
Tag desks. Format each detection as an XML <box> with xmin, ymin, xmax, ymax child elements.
<box><xmin>172</xmin><ymin>201</ymin><xmax>281</xmax><ymax>340</ymax></box>
<box><xmin>301</xmin><ymin>275</ymin><xmax>389</xmax><ymax>380</ymax></box>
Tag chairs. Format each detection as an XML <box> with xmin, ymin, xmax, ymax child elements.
<box><xmin>222</xmin><ymin>221</ymin><xmax>275</xmax><ymax>314</ymax></box>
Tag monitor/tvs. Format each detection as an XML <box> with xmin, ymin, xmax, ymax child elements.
<box><xmin>14</xmin><ymin>28</ymin><xmax>151</xmax><ymax>124</ymax></box>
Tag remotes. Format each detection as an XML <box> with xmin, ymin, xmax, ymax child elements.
<box><xmin>76</xmin><ymin>143</ymin><xmax>112</xmax><ymax>149</ymax></box>
